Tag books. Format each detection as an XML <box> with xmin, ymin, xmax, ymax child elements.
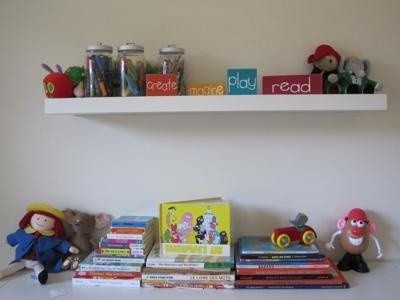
<box><xmin>71</xmin><ymin>195</ymin><xmax>350</xmax><ymax>289</ymax></box>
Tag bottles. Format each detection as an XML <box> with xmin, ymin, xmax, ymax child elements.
<box><xmin>158</xmin><ymin>45</ymin><xmax>184</xmax><ymax>93</ymax></box>
<box><xmin>116</xmin><ymin>43</ymin><xmax>145</xmax><ymax>98</ymax></box>
<box><xmin>84</xmin><ymin>43</ymin><xmax>114</xmax><ymax>97</ymax></box>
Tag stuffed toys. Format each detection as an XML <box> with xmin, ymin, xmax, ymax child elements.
<box><xmin>325</xmin><ymin>207</ymin><xmax>383</xmax><ymax>273</ymax></box>
<box><xmin>327</xmin><ymin>55</ymin><xmax>384</xmax><ymax>94</ymax></box>
<box><xmin>61</xmin><ymin>207</ymin><xmax>111</xmax><ymax>270</ymax></box>
<box><xmin>307</xmin><ymin>44</ymin><xmax>341</xmax><ymax>94</ymax></box>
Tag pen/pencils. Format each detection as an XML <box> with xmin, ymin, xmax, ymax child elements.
<box><xmin>158</xmin><ymin>55</ymin><xmax>185</xmax><ymax>95</ymax></box>
<box><xmin>115</xmin><ymin>55</ymin><xmax>144</xmax><ymax>96</ymax></box>
<box><xmin>41</xmin><ymin>63</ymin><xmax>54</xmax><ymax>73</ymax></box>
<box><xmin>56</xmin><ymin>64</ymin><xmax>63</xmax><ymax>73</ymax></box>
<box><xmin>82</xmin><ymin>54</ymin><xmax>113</xmax><ymax>97</ymax></box>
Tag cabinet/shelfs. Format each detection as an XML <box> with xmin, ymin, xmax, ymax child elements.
<box><xmin>1</xmin><ymin>92</ymin><xmax>399</xmax><ymax>300</ymax></box>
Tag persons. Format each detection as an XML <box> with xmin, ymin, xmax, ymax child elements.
<box><xmin>0</xmin><ymin>202</ymin><xmax>80</xmax><ymax>286</ymax></box>
